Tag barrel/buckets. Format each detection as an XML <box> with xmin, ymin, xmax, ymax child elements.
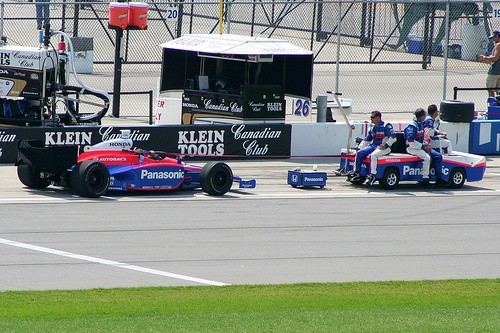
<box><xmin>68</xmin><ymin>37</ymin><xmax>93</xmax><ymax>74</ymax></box>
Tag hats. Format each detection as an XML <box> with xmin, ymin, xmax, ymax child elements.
<box><xmin>488</xmin><ymin>30</ymin><xmax>500</xmax><ymax>39</ymax></box>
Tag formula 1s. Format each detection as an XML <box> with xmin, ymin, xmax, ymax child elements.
<box><xmin>15</xmin><ymin>136</ymin><xmax>256</xmax><ymax>199</ymax></box>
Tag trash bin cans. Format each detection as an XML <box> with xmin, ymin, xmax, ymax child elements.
<box><xmin>451</xmin><ymin>44</ymin><xmax>461</xmax><ymax>58</ymax></box>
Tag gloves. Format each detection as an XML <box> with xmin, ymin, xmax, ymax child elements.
<box><xmin>475</xmin><ymin>54</ymin><xmax>485</xmax><ymax>61</ymax></box>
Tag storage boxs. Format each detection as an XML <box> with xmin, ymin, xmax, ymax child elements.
<box><xmin>409</xmin><ymin>39</ymin><xmax>424</xmax><ymax>54</ymax></box>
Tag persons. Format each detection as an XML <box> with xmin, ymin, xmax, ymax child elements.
<box><xmin>477</xmin><ymin>30</ymin><xmax>500</xmax><ymax>98</ymax></box>
<box><xmin>404</xmin><ymin>108</ymin><xmax>445</xmax><ymax>187</ymax></box>
<box><xmin>423</xmin><ymin>104</ymin><xmax>452</xmax><ymax>154</ymax></box>
<box><xmin>346</xmin><ymin>110</ymin><xmax>397</xmax><ymax>185</ymax></box>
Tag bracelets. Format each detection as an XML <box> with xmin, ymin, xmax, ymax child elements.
<box><xmin>482</xmin><ymin>56</ymin><xmax>486</xmax><ymax>62</ymax></box>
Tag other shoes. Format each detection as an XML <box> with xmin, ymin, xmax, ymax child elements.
<box><xmin>423</xmin><ymin>183</ymin><xmax>436</xmax><ymax>190</ymax></box>
<box><xmin>350</xmin><ymin>174</ymin><xmax>366</xmax><ymax>183</ymax></box>
<box><xmin>362</xmin><ymin>175</ymin><xmax>376</xmax><ymax>187</ymax></box>
<box><xmin>436</xmin><ymin>180</ymin><xmax>451</xmax><ymax>189</ymax></box>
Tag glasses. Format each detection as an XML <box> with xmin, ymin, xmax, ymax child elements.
<box><xmin>370</xmin><ymin>115</ymin><xmax>378</xmax><ymax>119</ymax></box>
